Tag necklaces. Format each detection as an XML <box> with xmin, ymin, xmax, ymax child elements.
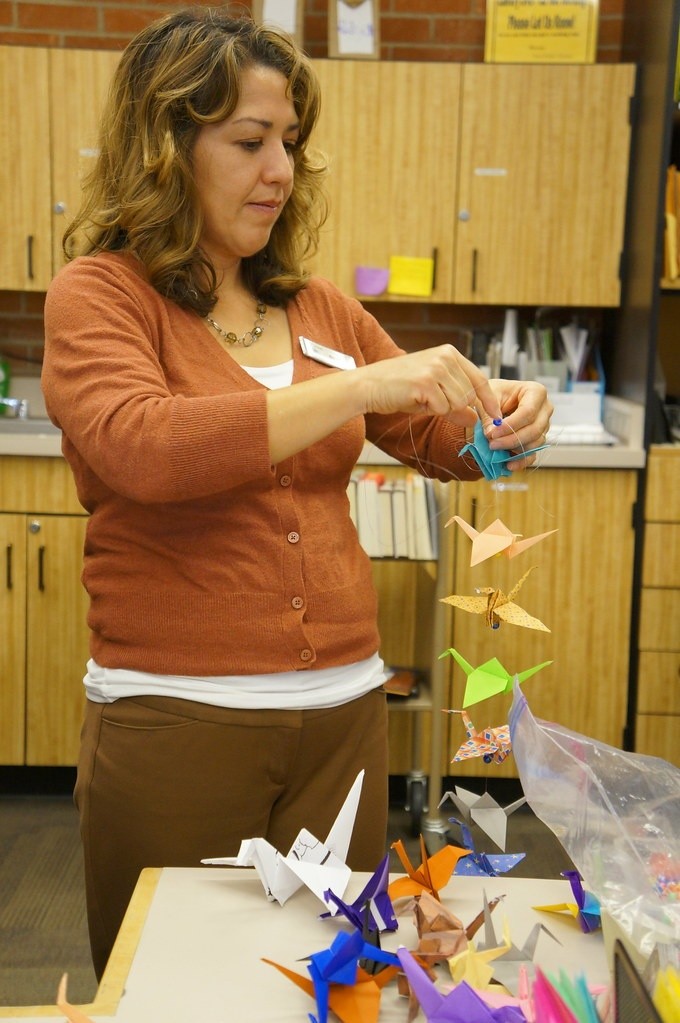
<box><xmin>198</xmin><ymin>303</ymin><xmax>270</xmax><ymax>348</ymax></box>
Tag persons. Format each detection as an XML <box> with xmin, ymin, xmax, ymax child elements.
<box><xmin>41</xmin><ymin>9</ymin><xmax>557</xmax><ymax>992</ymax></box>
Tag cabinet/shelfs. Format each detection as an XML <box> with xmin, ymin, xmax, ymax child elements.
<box><xmin>337</xmin><ymin>472</ymin><xmax>464</xmax><ymax>859</ymax></box>
<box><xmin>0</xmin><ymin>45</ymin><xmax>637</xmax><ymax>308</ymax></box>
<box><xmin>0</xmin><ymin>447</ymin><xmax>640</xmax><ymax>777</ymax></box>
<box><xmin>606</xmin><ymin>1</ymin><xmax>679</xmax><ymax>771</ymax></box>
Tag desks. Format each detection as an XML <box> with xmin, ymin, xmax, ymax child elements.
<box><xmin>0</xmin><ymin>867</ymin><xmax>619</xmax><ymax>1023</ymax></box>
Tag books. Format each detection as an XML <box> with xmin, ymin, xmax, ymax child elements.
<box><xmin>345</xmin><ymin>467</ymin><xmax>441</xmax><ymax>561</ymax></box>
